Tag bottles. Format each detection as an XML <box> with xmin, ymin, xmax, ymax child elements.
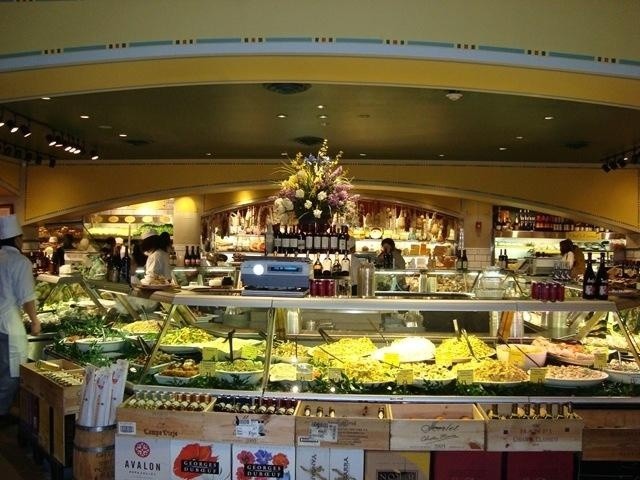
<box><xmin>169</xmin><ymin>252</ymin><xmax>173</xmax><ymax>266</ymax></box>
<box><xmin>316</xmin><ymin>405</ymin><xmax>324</xmax><ymax>418</ymax></box>
<box><xmin>214</xmin><ymin>394</ymin><xmax>299</xmax><ymax>414</ymax></box>
<box><xmin>498</xmin><ymin>248</ymin><xmax>502</xmax><ymax>269</ymax></box>
<box><xmin>357</xmin><ymin>256</ymin><xmax>375</xmax><ymax>297</ymax></box>
<box><xmin>532</xmin><ymin>279</ymin><xmax>564</xmax><ymax>302</ymax></box>
<box><xmin>463</xmin><ymin>249</ymin><xmax>468</xmax><ymax>269</ymax></box>
<box><xmin>173</xmin><ymin>252</ymin><xmax>177</xmax><ymax>266</ymax></box>
<box><xmin>582</xmin><ymin>251</ymin><xmax>596</xmax><ymax>300</ymax></box>
<box><xmin>457</xmin><ymin>249</ymin><xmax>462</xmax><ymax>270</ymax></box>
<box><xmin>48</xmin><ymin>237</ymin><xmax>59</xmax><ymax>276</ymax></box>
<box><xmin>496</xmin><ymin>209</ymin><xmax>609</xmax><ymax>233</ymax></box>
<box><xmin>428</xmin><ymin>253</ymin><xmax>432</xmax><ymax>270</ymax></box>
<box><xmin>328</xmin><ymin>406</ymin><xmax>336</xmax><ymax>418</ymax></box>
<box><xmin>432</xmin><ymin>254</ymin><xmax>436</xmax><ymax>269</ymax></box>
<box><xmin>128</xmin><ymin>389</ymin><xmax>210</xmax><ymax>412</ymax></box>
<box><xmin>183</xmin><ymin>244</ymin><xmax>201</xmax><ymax>267</ymax></box>
<box><xmin>484</xmin><ymin>400</ymin><xmax>576</xmax><ymax>419</ymax></box>
<box><xmin>265</xmin><ymin>250</ymin><xmax>350</xmax><ymax>278</ymax></box>
<box><xmin>503</xmin><ymin>249</ymin><xmax>508</xmax><ymax>268</ymax></box>
<box><xmin>596</xmin><ymin>252</ymin><xmax>609</xmax><ymax>302</ymax></box>
<box><xmin>28</xmin><ymin>249</ymin><xmax>46</xmax><ymax>272</ymax></box>
<box><xmin>303</xmin><ymin>404</ymin><xmax>311</xmax><ymax>417</ymax></box>
<box><xmin>377</xmin><ymin>406</ymin><xmax>385</xmax><ymax>419</ymax></box>
<box><xmin>36</xmin><ymin>358</ymin><xmax>61</xmax><ymax>372</ymax></box>
<box><xmin>109</xmin><ymin>244</ymin><xmax>130</xmax><ymax>285</ymax></box>
<box><xmin>265</xmin><ymin>224</ymin><xmax>356</xmax><ymax>254</ymax></box>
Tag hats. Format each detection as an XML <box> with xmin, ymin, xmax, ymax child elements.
<box><xmin>141</xmin><ymin>231</ymin><xmax>158</xmax><ymax>240</ymax></box>
<box><xmin>0</xmin><ymin>215</ymin><xmax>23</xmax><ymax>239</ymax></box>
<box><xmin>49</xmin><ymin>237</ymin><xmax>58</xmax><ymax>242</ymax></box>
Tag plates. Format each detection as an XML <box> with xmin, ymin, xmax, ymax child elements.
<box><xmin>123</xmin><ymin>362</ymin><xmax>178</xmax><ymax>374</ymax></box>
<box><xmin>369</xmin><ymin>226</ymin><xmax>384</xmax><ymax>239</ymax></box>
<box><xmin>125</xmin><ymin>334</ymin><xmax>156</xmax><ymax>350</ymax></box>
<box><xmin>463</xmin><ymin>379</ymin><xmax>529</xmax><ymax>389</ymax></box>
<box><xmin>158</xmin><ymin>344</ymin><xmax>196</xmax><ymax>355</ymax></box>
<box><xmin>454</xmin><ymin>349</ymin><xmax>496</xmax><ymax>363</ymax></box>
<box><xmin>404</xmin><ymin>376</ymin><xmax>457</xmax><ymax>392</ymax></box>
<box><xmin>526</xmin><ymin>365</ymin><xmax>609</xmax><ymax>389</ymax></box>
<box><xmin>351</xmin><ymin>381</ymin><xmax>387</xmax><ymax>390</ymax></box>
<box><xmin>153</xmin><ymin>310</ymin><xmax>220</xmax><ymax>323</ymax></box>
<box><xmin>154</xmin><ymin>373</ymin><xmax>200</xmax><ymax>385</ymax></box>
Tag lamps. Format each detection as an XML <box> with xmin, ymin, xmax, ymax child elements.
<box><xmin>600</xmin><ymin>145</ymin><xmax>640</xmax><ymax>173</ymax></box>
<box><xmin>0</xmin><ymin>105</ymin><xmax>103</xmax><ymax>169</ymax></box>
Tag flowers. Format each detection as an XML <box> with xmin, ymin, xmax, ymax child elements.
<box><xmin>265</xmin><ymin>137</ymin><xmax>364</xmax><ymax>230</ymax></box>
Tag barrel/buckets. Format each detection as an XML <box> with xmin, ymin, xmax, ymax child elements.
<box><xmin>72</xmin><ymin>422</ymin><xmax>116</xmax><ymax>480</ymax></box>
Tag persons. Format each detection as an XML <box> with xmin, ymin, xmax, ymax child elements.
<box><xmin>373</xmin><ymin>238</ymin><xmax>409</xmax><ymax>290</ymax></box>
<box><xmin>48</xmin><ymin>234</ymin><xmax>78</xmax><ymax>271</ymax></box>
<box><xmin>0</xmin><ymin>214</ymin><xmax>42</xmax><ymax>420</ymax></box>
<box><xmin>558</xmin><ymin>238</ymin><xmax>586</xmax><ymax>280</ymax></box>
<box><xmin>105</xmin><ymin>229</ymin><xmax>171</xmax><ymax>286</ymax></box>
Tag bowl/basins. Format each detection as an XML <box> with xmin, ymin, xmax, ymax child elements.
<box><xmin>223</xmin><ymin>312</ymin><xmax>251</xmax><ymax>328</ymax></box>
<box><xmin>113</xmin><ymin>293</ymin><xmax>159</xmax><ymax>315</ymax></box>
<box><xmin>604</xmin><ymin>369</ymin><xmax>640</xmax><ymax>384</ymax></box>
<box><xmin>495</xmin><ymin>344</ymin><xmax>547</xmax><ymax>370</ymax></box>
<box><xmin>76</xmin><ymin>338</ymin><xmax>125</xmax><ymax>354</ymax></box>
<box><xmin>213</xmin><ymin>369</ymin><xmax>264</xmax><ymax>389</ymax></box>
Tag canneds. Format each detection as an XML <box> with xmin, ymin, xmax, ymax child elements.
<box><xmin>356</xmin><ymin>262</ymin><xmax>375</xmax><ymax>298</ymax></box>
